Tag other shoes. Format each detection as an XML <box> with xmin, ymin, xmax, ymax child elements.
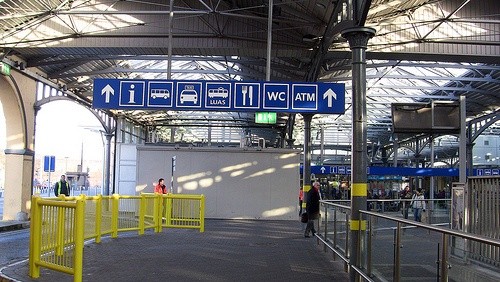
<box><xmin>305</xmin><ymin>236</ymin><xmax>309</xmax><ymax>237</ymax></box>
<box><xmin>313</xmin><ymin>234</ymin><xmax>315</xmax><ymax>236</ymax></box>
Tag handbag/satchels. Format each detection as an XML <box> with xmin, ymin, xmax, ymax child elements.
<box><xmin>301</xmin><ymin>213</ymin><xmax>308</xmax><ymax>223</ymax></box>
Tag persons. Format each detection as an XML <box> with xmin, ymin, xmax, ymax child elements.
<box><xmin>437</xmin><ymin>189</ymin><xmax>445</xmax><ymax>209</ymax></box>
<box><xmin>54</xmin><ymin>175</ymin><xmax>71</xmax><ymax>197</ymax></box>
<box><xmin>401</xmin><ymin>186</ymin><xmax>426</xmax><ymax>222</ymax></box>
<box><xmin>155</xmin><ymin>178</ymin><xmax>168</xmax><ymax>225</ymax></box>
<box><xmin>299</xmin><ymin>181</ymin><xmax>323</xmax><ymax>238</ymax></box>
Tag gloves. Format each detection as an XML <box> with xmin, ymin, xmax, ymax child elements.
<box><xmin>423</xmin><ymin>210</ymin><xmax>425</xmax><ymax>212</ymax></box>
